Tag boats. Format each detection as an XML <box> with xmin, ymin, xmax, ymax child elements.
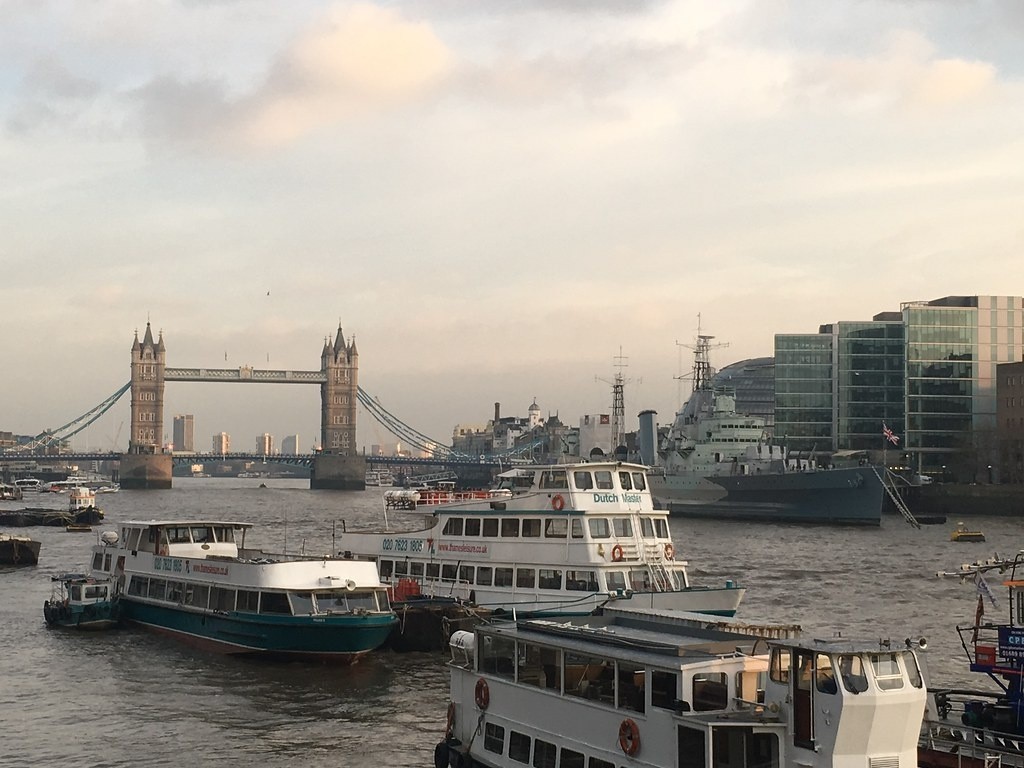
<box><xmin>69</xmin><ymin>487</ymin><xmax>105</xmax><ymax>523</ymax></box>
<box><xmin>949</xmin><ymin>526</ymin><xmax>985</xmax><ymax>542</ymax></box>
<box><xmin>66</xmin><ymin>524</ymin><xmax>92</xmax><ymax>533</ymax></box>
<box><xmin>43</xmin><ymin>473</ymin><xmax>120</xmax><ymax>494</ymax></box>
<box><xmin>89</xmin><ymin>520</ymin><xmax>400</xmax><ymax>665</ymax></box>
<box><xmin>938</xmin><ymin>549</ymin><xmax>1024</xmax><ymax>735</ymax></box>
<box><xmin>906</xmin><ymin>516</ymin><xmax>947</xmax><ymax>525</ymax></box>
<box><xmin>193</xmin><ymin>472</ymin><xmax>212</xmax><ymax>479</ymax></box>
<box><xmin>340</xmin><ymin>462</ymin><xmax>746</xmax><ymax>616</ymax></box>
<box><xmin>44</xmin><ymin>574</ymin><xmax>121</xmax><ymax>633</ymax></box>
<box><xmin>432</xmin><ymin>606</ymin><xmax>1023</xmax><ymax>767</ymax></box>
<box><xmin>576</xmin><ymin>314</ymin><xmax>883</xmax><ymax>527</ymax></box>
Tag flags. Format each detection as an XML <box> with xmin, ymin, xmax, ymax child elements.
<box><xmin>971</xmin><ymin>597</ymin><xmax>984</xmax><ymax>642</ymax></box>
<box><xmin>883</xmin><ymin>424</ymin><xmax>899</xmax><ymax>445</ymax></box>
<box><xmin>975</xmin><ymin>572</ymin><xmax>1001</xmax><ymax>609</ymax></box>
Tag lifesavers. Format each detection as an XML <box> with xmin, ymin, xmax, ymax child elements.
<box><xmin>612</xmin><ymin>545</ymin><xmax>623</xmax><ymax>562</ymax></box>
<box><xmin>552</xmin><ymin>494</ymin><xmax>564</xmax><ymax>511</ymax></box>
<box><xmin>619</xmin><ymin>717</ymin><xmax>640</xmax><ymax>755</ymax></box>
<box><xmin>665</xmin><ymin>545</ymin><xmax>675</xmax><ymax>560</ymax></box>
<box><xmin>434</xmin><ymin>742</ymin><xmax>449</xmax><ymax>768</ymax></box>
<box><xmin>474</xmin><ymin>678</ymin><xmax>490</xmax><ymax>710</ymax></box>
